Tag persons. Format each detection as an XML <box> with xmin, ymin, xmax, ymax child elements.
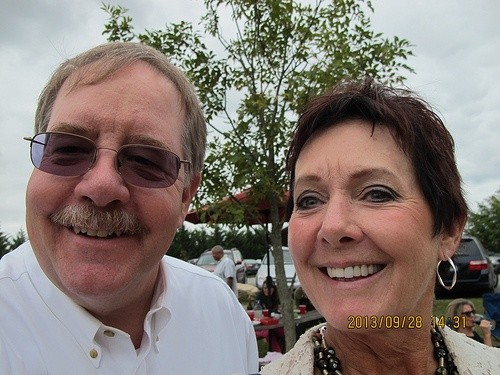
<box><xmin>258</xmin><ymin>280</ymin><xmax>279</xmax><ymax>310</ymax></box>
<box><xmin>447</xmin><ymin>299</ymin><xmax>492</xmax><ymax>349</ymax></box>
<box><xmin>260</xmin><ymin>77</ymin><xmax>500</xmax><ymax>375</ymax></box>
<box><xmin>0</xmin><ymin>43</ymin><xmax>259</xmax><ymax>375</ymax></box>
<box><xmin>212</xmin><ymin>245</ymin><xmax>237</xmax><ymax>300</ymax></box>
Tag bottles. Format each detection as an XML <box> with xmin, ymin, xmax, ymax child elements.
<box><xmin>247</xmin><ymin>300</ymin><xmax>253</xmax><ymax>313</ymax></box>
<box><xmin>253</xmin><ymin>300</ymin><xmax>262</xmax><ymax>321</ymax></box>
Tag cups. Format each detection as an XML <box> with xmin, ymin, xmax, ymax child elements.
<box><xmin>299</xmin><ymin>305</ymin><xmax>306</xmax><ymax>314</ymax></box>
<box><xmin>247</xmin><ymin>311</ymin><xmax>255</xmax><ymax>321</ymax></box>
<box><xmin>262</xmin><ymin>310</ymin><xmax>269</xmax><ymax>319</ymax></box>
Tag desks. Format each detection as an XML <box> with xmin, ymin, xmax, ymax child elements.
<box><xmin>253</xmin><ymin>310</ymin><xmax>324</xmax><ymax>349</ymax></box>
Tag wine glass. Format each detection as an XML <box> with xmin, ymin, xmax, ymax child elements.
<box><xmin>472</xmin><ymin>314</ymin><xmax>495</xmax><ymax>330</ymax></box>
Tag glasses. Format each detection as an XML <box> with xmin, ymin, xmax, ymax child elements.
<box><xmin>460</xmin><ymin>309</ymin><xmax>477</xmax><ymax>318</ymax></box>
<box><xmin>24</xmin><ymin>132</ymin><xmax>191</xmax><ymax>190</ymax></box>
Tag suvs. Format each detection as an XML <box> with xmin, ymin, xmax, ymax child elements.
<box><xmin>434</xmin><ymin>237</ymin><xmax>498</xmax><ymax>298</ymax></box>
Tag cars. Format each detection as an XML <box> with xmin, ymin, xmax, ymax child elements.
<box><xmin>489</xmin><ymin>257</ymin><xmax>500</xmax><ymax>275</ymax></box>
<box><xmin>256</xmin><ymin>245</ymin><xmax>301</xmax><ymax>293</ymax></box>
<box><xmin>187</xmin><ymin>247</ymin><xmax>261</xmax><ymax>284</ymax></box>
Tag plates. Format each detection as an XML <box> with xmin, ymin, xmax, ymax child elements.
<box><xmin>253</xmin><ymin>321</ymin><xmax>261</xmax><ymax>326</ymax></box>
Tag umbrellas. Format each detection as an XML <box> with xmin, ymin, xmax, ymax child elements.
<box><xmin>186</xmin><ymin>181</ymin><xmax>292</xmax><ymax>275</ymax></box>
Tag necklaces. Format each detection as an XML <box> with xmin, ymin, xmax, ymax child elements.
<box><xmin>312</xmin><ymin>325</ymin><xmax>459</xmax><ymax>375</ymax></box>
